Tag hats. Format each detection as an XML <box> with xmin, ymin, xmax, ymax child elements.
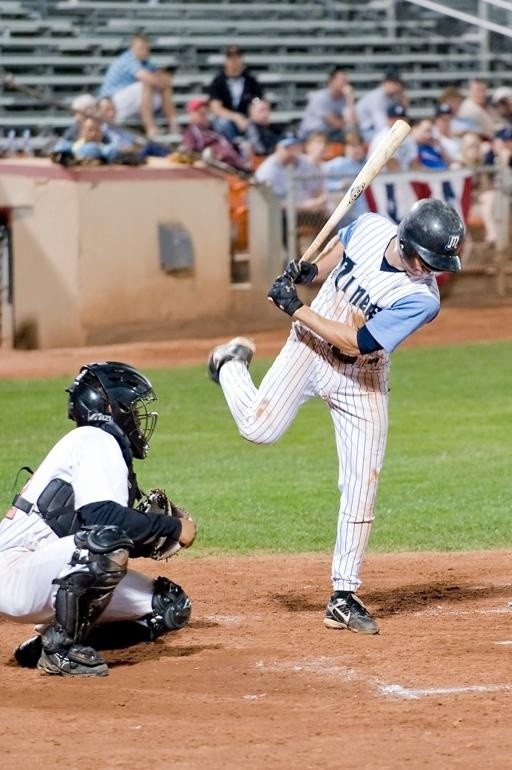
<box><xmin>492</xmin><ymin>85</ymin><xmax>511</xmax><ymax>103</ymax></box>
<box><xmin>228</xmin><ymin>44</ymin><xmax>244</xmax><ymax>57</ymax></box>
<box><xmin>383</xmin><ymin>65</ymin><xmax>407</xmax><ymax>86</ymax></box>
<box><xmin>276</xmin><ymin>126</ymin><xmax>303</xmax><ymax>148</ymax></box>
<box><xmin>438</xmin><ymin>101</ymin><xmax>452</xmax><ymax>113</ymax></box>
<box><xmin>495</xmin><ymin>127</ymin><xmax>512</xmax><ymax>140</ymax></box>
<box><xmin>186</xmin><ymin>94</ymin><xmax>209</xmax><ymax>109</ymax></box>
<box><xmin>72</xmin><ymin>93</ymin><xmax>96</xmax><ymax>110</ymax></box>
<box><xmin>389</xmin><ymin>103</ymin><xmax>406</xmax><ymax>118</ymax></box>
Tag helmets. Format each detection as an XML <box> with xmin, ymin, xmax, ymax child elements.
<box><xmin>65</xmin><ymin>358</ymin><xmax>155</xmax><ymax>456</ymax></box>
<box><xmin>396</xmin><ymin>198</ymin><xmax>463</xmax><ymax>275</ymax></box>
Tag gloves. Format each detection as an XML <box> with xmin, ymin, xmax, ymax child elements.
<box><xmin>267</xmin><ymin>258</ymin><xmax>319</xmax><ymax>317</ymax></box>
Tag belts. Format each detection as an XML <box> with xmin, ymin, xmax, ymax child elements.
<box><xmin>326</xmin><ymin>341</ymin><xmax>389</xmax><ymax>366</ymax></box>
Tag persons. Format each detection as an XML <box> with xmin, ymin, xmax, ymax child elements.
<box><xmin>52</xmin><ymin>30</ymin><xmax>512</xmax><ymax>259</ymax></box>
<box><xmin>1</xmin><ymin>361</ymin><xmax>197</xmax><ymax>682</ymax></box>
<box><xmin>205</xmin><ymin>197</ymin><xmax>465</xmax><ymax>636</ymax></box>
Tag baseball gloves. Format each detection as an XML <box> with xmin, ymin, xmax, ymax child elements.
<box><xmin>128</xmin><ymin>491</ymin><xmax>193</xmax><ymax>561</ymax></box>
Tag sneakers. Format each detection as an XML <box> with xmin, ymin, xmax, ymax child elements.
<box><xmin>204</xmin><ymin>336</ymin><xmax>255</xmax><ymax>380</ymax></box>
<box><xmin>322</xmin><ymin>593</ymin><xmax>379</xmax><ymax>635</ymax></box>
<box><xmin>12</xmin><ymin>634</ymin><xmax>108</xmax><ymax>674</ymax></box>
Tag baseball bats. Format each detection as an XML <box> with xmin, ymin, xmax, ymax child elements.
<box><xmin>267</xmin><ymin>119</ymin><xmax>411</xmax><ymax>303</ymax></box>
